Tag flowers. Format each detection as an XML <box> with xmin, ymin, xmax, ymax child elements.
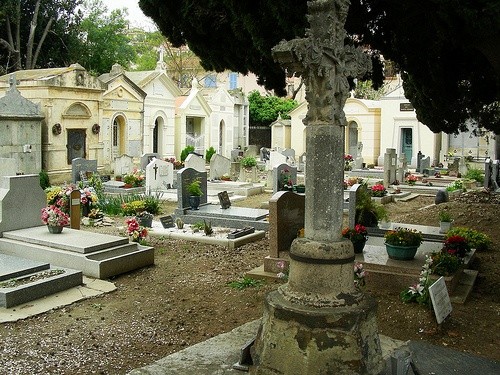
<box><xmin>164</xmin><ymin>158</ymin><xmax>181</xmax><ymax>168</ymax></box>
<box><xmin>41</xmin><ymin>205</ymin><xmax>69</xmax><ymax>227</ymax></box>
<box><xmin>343</xmin><ymin>154</ymin><xmax>354</xmax><ymax>164</ymax></box>
<box><xmin>87</xmin><ymin>208</ymin><xmax>103</xmax><ymax>218</ymax></box>
<box><xmin>371</xmin><ymin>183</ymin><xmax>388</xmax><ymax>197</ymax></box>
<box><xmin>383</xmin><ymin>226</ymin><xmax>424</xmax><ymax>246</ymax></box>
<box><xmin>44</xmin><ymin>185</ymin><xmax>100</xmax><ymax>207</ymax></box>
<box><xmin>400</xmin><ymin>236</ymin><xmax>470</xmax><ymax>305</ymax></box>
<box><xmin>284</xmin><ymin>180</ymin><xmax>293</xmax><ymax>188</ymax></box>
<box><xmin>405</xmin><ymin>174</ymin><xmax>418</xmax><ymax>185</ymax></box>
<box><xmin>124</xmin><ymin>176</ymin><xmax>144</xmax><ymax>184</ymax></box>
<box><xmin>126</xmin><ymin>219</ymin><xmax>148</xmax><ymax>239</ymax></box>
<box><xmin>342</xmin><ymin>224</ymin><xmax>369</xmax><ymax>243</ymax></box>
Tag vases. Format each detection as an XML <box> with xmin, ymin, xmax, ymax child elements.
<box><xmin>384</xmin><ymin>242</ymin><xmax>419</xmax><ymax>260</ymax></box>
<box><xmin>46</xmin><ymin>225</ymin><xmax>63</xmax><ymax>233</ymax></box>
<box><xmin>352</xmin><ymin>240</ymin><xmax>365</xmax><ymax>253</ymax></box>
<box><xmin>130</xmin><ymin>234</ymin><xmax>138</xmax><ymax>242</ymax></box>
<box><xmin>91</xmin><ymin>218</ymin><xmax>103</xmax><ymax>227</ymax></box>
<box><xmin>125</xmin><ymin>183</ymin><xmax>131</xmax><ymax>188</ymax></box>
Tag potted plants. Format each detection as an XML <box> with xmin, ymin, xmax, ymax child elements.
<box><xmin>187</xmin><ymin>181</ymin><xmax>204</xmax><ymax>210</ymax></box>
<box><xmin>115</xmin><ymin>175</ymin><xmax>122</xmax><ymax>181</ymax></box>
<box><xmin>297</xmin><ymin>184</ymin><xmax>305</xmax><ymax>193</ymax></box>
